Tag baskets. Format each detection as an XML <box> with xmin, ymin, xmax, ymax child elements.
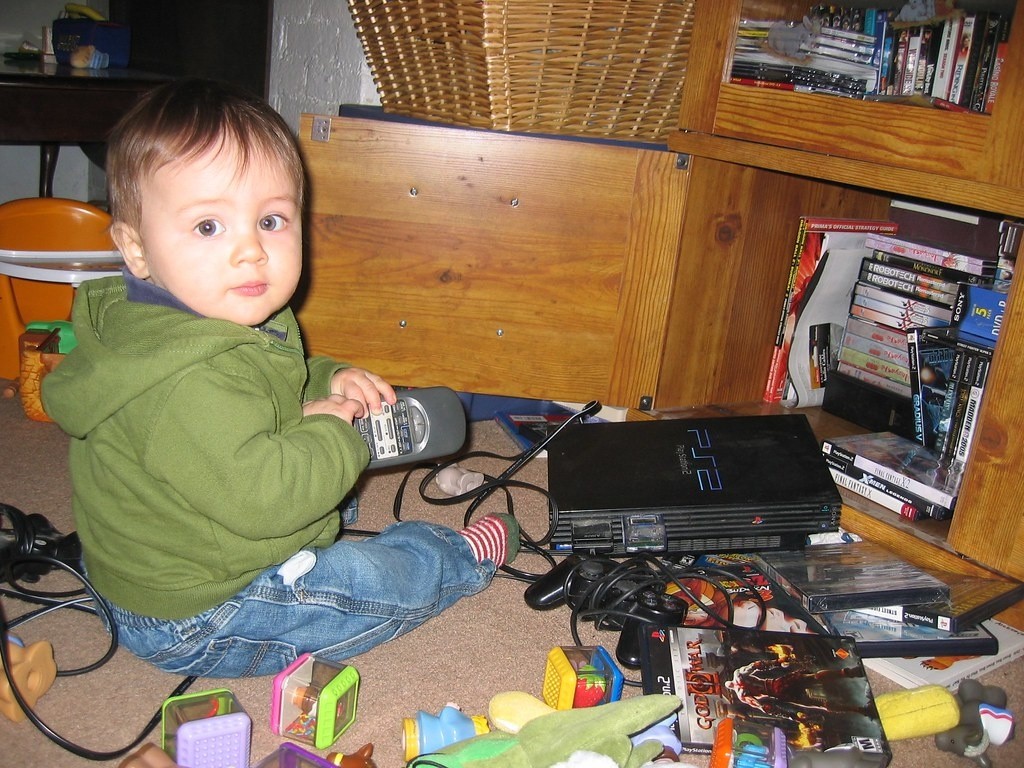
<box><xmin>347</xmin><ymin>0</ymin><xmax>698</xmax><ymax>140</ymax></box>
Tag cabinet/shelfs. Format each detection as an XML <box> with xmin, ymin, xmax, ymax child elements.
<box><xmin>294</xmin><ymin>1</ymin><xmax>1024</xmax><ymax>632</ymax></box>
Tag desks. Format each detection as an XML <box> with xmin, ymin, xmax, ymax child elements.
<box><xmin>0</xmin><ymin>52</ymin><xmax>189</xmax><ymax>199</ymax></box>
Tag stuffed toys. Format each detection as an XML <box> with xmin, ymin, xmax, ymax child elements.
<box><xmin>874</xmin><ymin>679</ymin><xmax>1016</xmax><ymax>768</ymax></box>
<box><xmin>405</xmin><ymin>694</ymin><xmax>682</xmax><ymax>768</ymax></box>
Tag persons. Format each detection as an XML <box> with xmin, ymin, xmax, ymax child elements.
<box><xmin>899</xmin><ymin>30</ymin><xmax>908</xmax><ymax>42</ymax></box>
<box><xmin>40</xmin><ymin>78</ymin><xmax>520</xmax><ymax>678</ymax></box>
<box><xmin>962</xmin><ymin>34</ymin><xmax>971</xmax><ymax>51</ymax></box>
<box><xmin>833</xmin><ymin>17</ymin><xmax>840</xmax><ymax>27</ymax></box>
<box><xmin>843</xmin><ymin>19</ymin><xmax>849</xmax><ymax>29</ymax></box>
<box><xmin>854</xmin><ymin>18</ymin><xmax>860</xmax><ymax>30</ymax></box>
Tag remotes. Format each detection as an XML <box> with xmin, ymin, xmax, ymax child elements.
<box><xmin>351</xmin><ymin>386</ymin><xmax>467</xmax><ymax>470</ymax></box>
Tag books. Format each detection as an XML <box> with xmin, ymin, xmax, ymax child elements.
<box><xmin>863</xmin><ymin>618</ymin><xmax>1024</xmax><ymax>689</ymax></box>
